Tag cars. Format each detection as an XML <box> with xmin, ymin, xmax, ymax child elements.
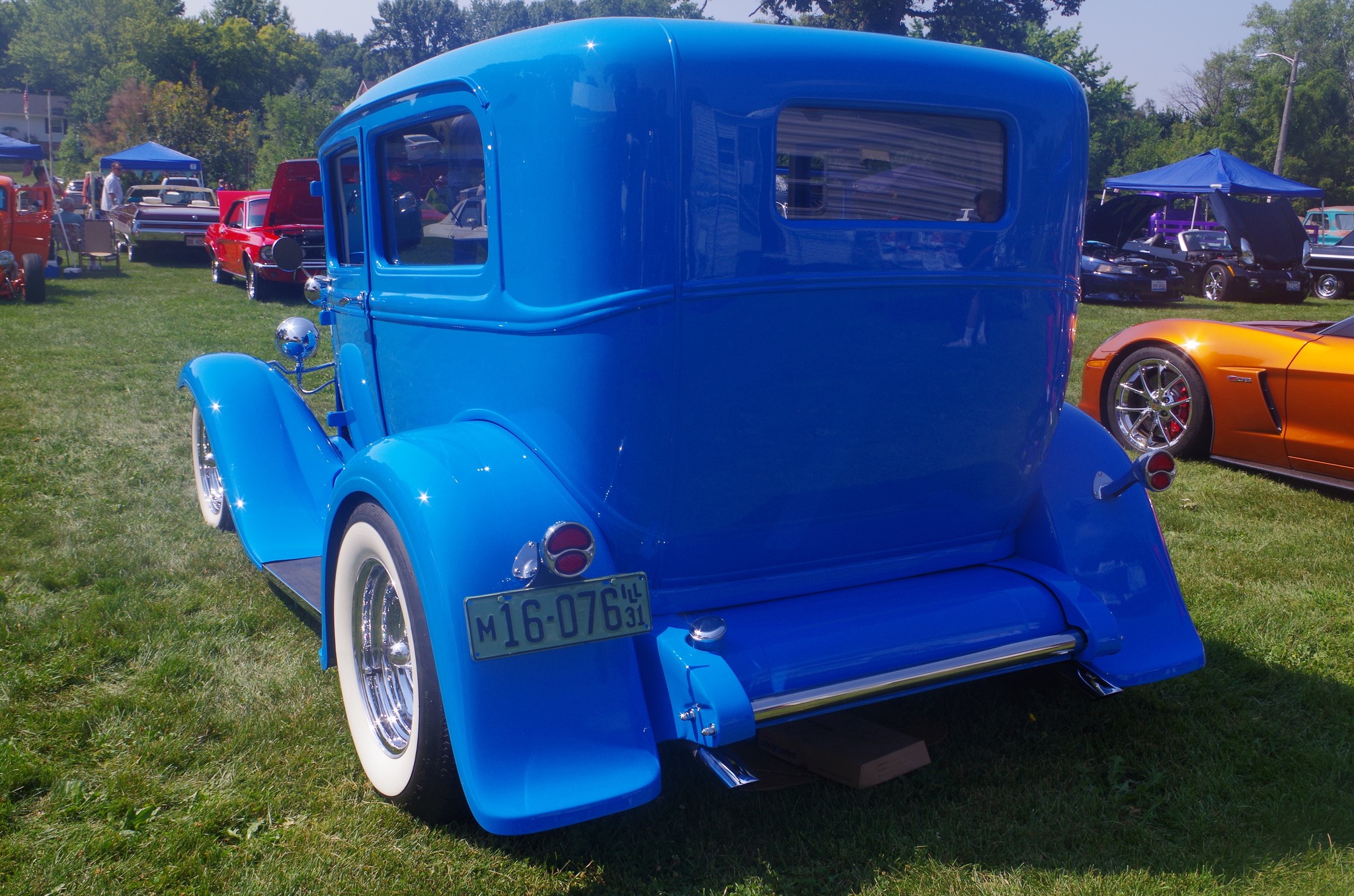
<box><xmin>175</xmin><ymin>13</ymin><xmax>1211</xmax><ymax>843</ymax></box>
<box><xmin>392</xmin><ymin>130</ymin><xmax>486</xmax><ymax>243</ymax></box>
<box><xmin>1077</xmin><ymin>308</ymin><xmax>1352</xmax><ymax>498</ymax></box>
<box><xmin>1074</xmin><ymin>203</ymin><xmax>1354</xmax><ymax>308</ymax></box>
<box><xmin>1</xmin><ymin>154</ymin><xmax>327</xmax><ymax>304</ymax></box>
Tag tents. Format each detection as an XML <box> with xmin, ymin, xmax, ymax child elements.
<box><xmin>1100</xmin><ymin>148</ymin><xmax>1325</xmax><ymax>245</ymax></box>
<box><xmin>100</xmin><ymin>141</ymin><xmax>206</xmax><ymax>202</ymax></box>
<box><xmin>0</xmin><ymin>133</ymin><xmax>77</xmax><ymax>273</ymax></box>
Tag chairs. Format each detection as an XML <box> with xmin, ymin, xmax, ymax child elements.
<box><xmin>77</xmin><ymin>218</ymin><xmax>121</xmax><ymax>277</ymax></box>
<box><xmin>51</xmin><ymin>222</ymin><xmax>103</xmax><ymax>268</ymax></box>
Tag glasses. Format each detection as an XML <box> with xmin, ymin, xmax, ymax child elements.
<box><xmin>114</xmin><ymin>168</ymin><xmax>123</xmax><ymax>170</ymax></box>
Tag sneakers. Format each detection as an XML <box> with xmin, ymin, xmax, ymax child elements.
<box><xmin>105</xmin><ymin>252</ymin><xmax>121</xmax><ymax>262</ymax></box>
<box><xmin>87</xmin><ymin>264</ymin><xmax>101</xmax><ymax>271</ymax></box>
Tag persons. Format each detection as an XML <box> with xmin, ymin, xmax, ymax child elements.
<box><xmin>217</xmin><ymin>179</ymin><xmax>225</xmax><ymax>190</ymax></box>
<box><xmin>476</xmin><ymin>180</ymin><xmax>488</xmax><ymax>252</ymax></box>
<box><xmin>224</xmin><ymin>182</ymin><xmax>231</xmax><ymax>190</ymax></box>
<box><xmin>424</xmin><ymin>175</ymin><xmax>454</xmax><ymax>252</ymax></box>
<box><xmin>941</xmin><ymin>188</ymin><xmax>1005</xmax><ymax>349</ymax></box>
<box><xmin>51</xmin><ymin>197</ymin><xmax>85</xmax><ymax>227</ymax></box>
<box><xmin>100</xmin><ymin>162</ymin><xmax>124</xmax><ymax>219</ymax></box>
<box><xmin>230</xmin><ymin>182</ymin><xmax>236</xmax><ymax>190</ymax></box>
<box><xmin>32</xmin><ymin>166</ymin><xmax>64</xmax><ymax>261</ymax></box>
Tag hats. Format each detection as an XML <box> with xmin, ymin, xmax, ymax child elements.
<box><xmin>480</xmin><ymin>172</ymin><xmax>485</xmax><ymax>182</ymax></box>
<box><xmin>434</xmin><ymin>176</ymin><xmax>448</xmax><ymax>186</ymax></box>
